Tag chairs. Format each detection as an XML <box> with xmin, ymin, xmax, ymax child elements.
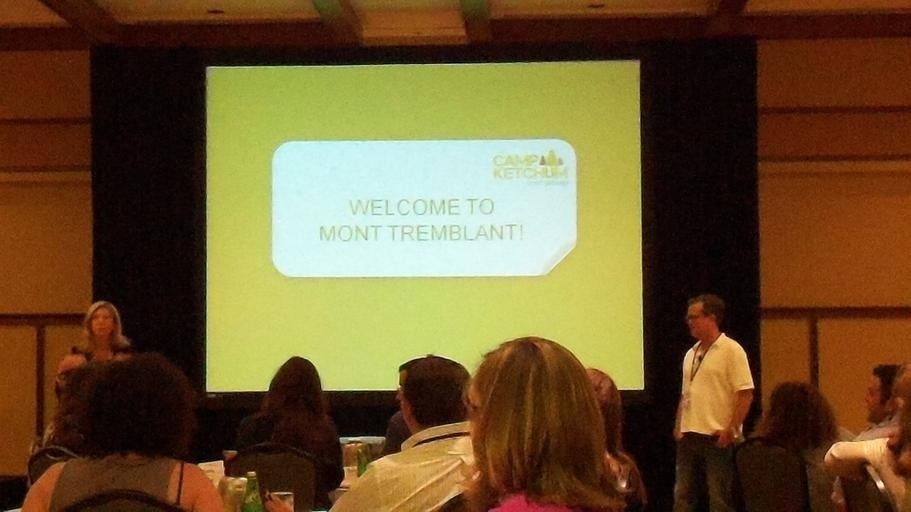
<box><xmin>26</xmin><ymin>436</ymin><xmax>897</xmax><ymax>512</ymax></box>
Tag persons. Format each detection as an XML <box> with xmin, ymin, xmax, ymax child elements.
<box><xmin>673</xmin><ymin>293</ymin><xmax>756</xmax><ymax>512</ymax></box>
<box><xmin>733</xmin><ymin>364</ymin><xmax>911</xmax><ymax>512</ymax></box>
<box><xmin>21</xmin><ymin>350</ymin><xmax>224</xmax><ymax>512</ymax></box>
<box><xmin>328</xmin><ymin>337</ymin><xmax>649</xmax><ymax>512</ymax></box>
<box><xmin>235</xmin><ymin>356</ymin><xmax>344</xmax><ymax>510</ymax></box>
<box><xmin>28</xmin><ymin>301</ymin><xmax>133</xmax><ymax>487</ymax></box>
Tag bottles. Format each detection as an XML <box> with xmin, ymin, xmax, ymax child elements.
<box><xmin>241</xmin><ymin>472</ymin><xmax>262</xmax><ymax>512</ymax></box>
